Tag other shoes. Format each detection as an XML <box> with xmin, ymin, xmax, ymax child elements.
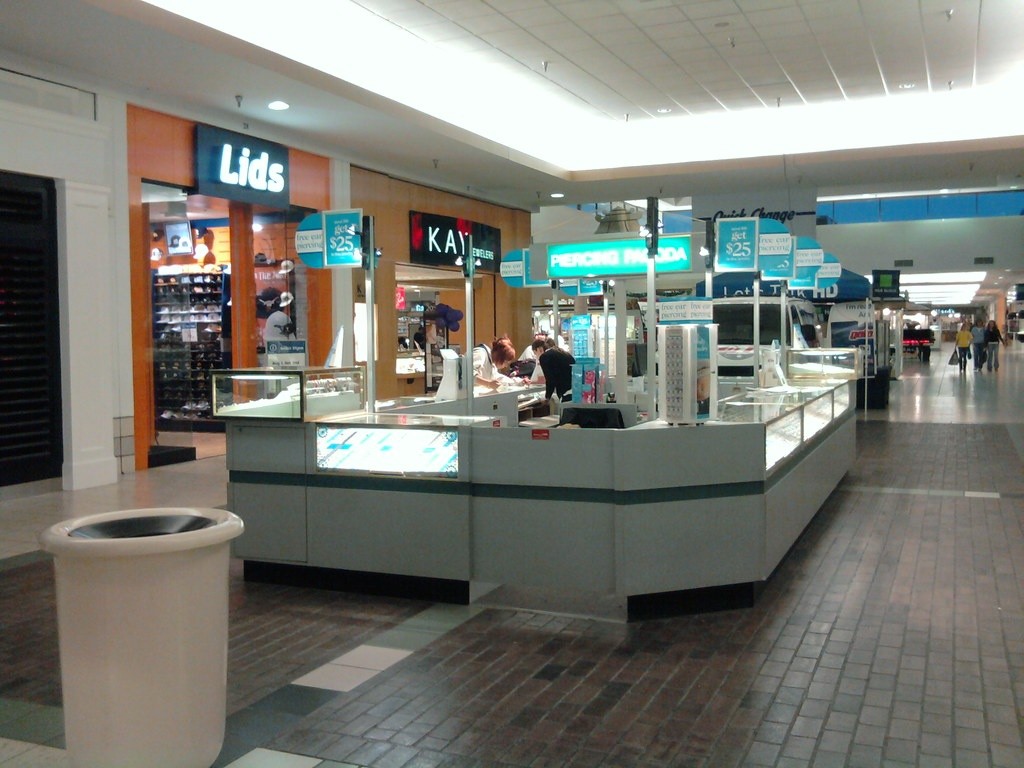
<box><xmin>959</xmin><ymin>368</ymin><xmax>1000</xmax><ymax>372</ymax></box>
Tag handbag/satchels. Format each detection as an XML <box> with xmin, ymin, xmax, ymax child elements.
<box><xmin>983</xmin><ymin>351</ymin><xmax>987</xmax><ymax>364</ymax></box>
<box><xmin>968</xmin><ymin>348</ymin><xmax>971</xmax><ymax>359</ymax></box>
<box><xmin>948</xmin><ymin>350</ymin><xmax>959</xmax><ymax>364</ymax></box>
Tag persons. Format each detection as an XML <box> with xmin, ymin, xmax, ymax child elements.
<box><xmin>955</xmin><ymin>323</ymin><xmax>973</xmax><ymax>371</ymax></box>
<box><xmin>984</xmin><ymin>320</ymin><xmax>1005</xmax><ymax>372</ymax></box>
<box><xmin>463</xmin><ymin>336</ymin><xmax>516</xmax><ymax>398</ymax></box>
<box><xmin>970</xmin><ymin>319</ymin><xmax>987</xmax><ymax>371</ymax></box>
<box><xmin>515</xmin><ymin>329</ymin><xmax>550</xmax><ymax>360</ymax></box>
<box><xmin>531</xmin><ymin>338</ymin><xmax>576</xmax><ymax>402</ymax></box>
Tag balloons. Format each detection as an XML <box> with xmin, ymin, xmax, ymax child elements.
<box><xmin>424</xmin><ymin>303</ymin><xmax>465</xmax><ymax>332</ymax></box>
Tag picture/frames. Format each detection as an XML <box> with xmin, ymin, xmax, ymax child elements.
<box><xmin>164</xmin><ymin>221</ymin><xmax>193</xmax><ymax>254</ymax></box>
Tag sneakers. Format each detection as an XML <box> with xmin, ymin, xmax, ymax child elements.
<box><xmin>160</xmin><ymin>400</ymin><xmax>229</xmax><ymax>421</ymax></box>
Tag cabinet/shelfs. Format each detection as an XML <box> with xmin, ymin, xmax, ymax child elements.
<box><xmin>395</xmin><ymin>350</ymin><xmax>425</xmax><ymax>374</ymax></box>
<box><xmin>618</xmin><ymin>377</ymin><xmax>857</xmax><ymax>606</ymax></box>
<box><xmin>224</xmin><ymin>415</ymin><xmax>503</xmax><ymax>602</ymax></box>
<box><xmin>367</xmin><ymin>379</ymin><xmax>554</xmax><ymax>426</ymax></box>
<box><xmin>210</xmin><ymin>364</ymin><xmax>367</xmax><ymax>420</ymax></box>
<box><xmin>150</xmin><ymin>264</ymin><xmax>232</xmax><ymax>433</ymax></box>
<box><xmin>787</xmin><ymin>343</ymin><xmax>862</xmax><ymax>379</ymax></box>
<box><xmin>398</xmin><ymin>309</ymin><xmax>424</xmax><ymax>337</ymax></box>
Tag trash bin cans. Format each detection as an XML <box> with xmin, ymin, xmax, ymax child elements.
<box><xmin>38</xmin><ymin>506</ymin><xmax>246</xmax><ymax>767</ymax></box>
